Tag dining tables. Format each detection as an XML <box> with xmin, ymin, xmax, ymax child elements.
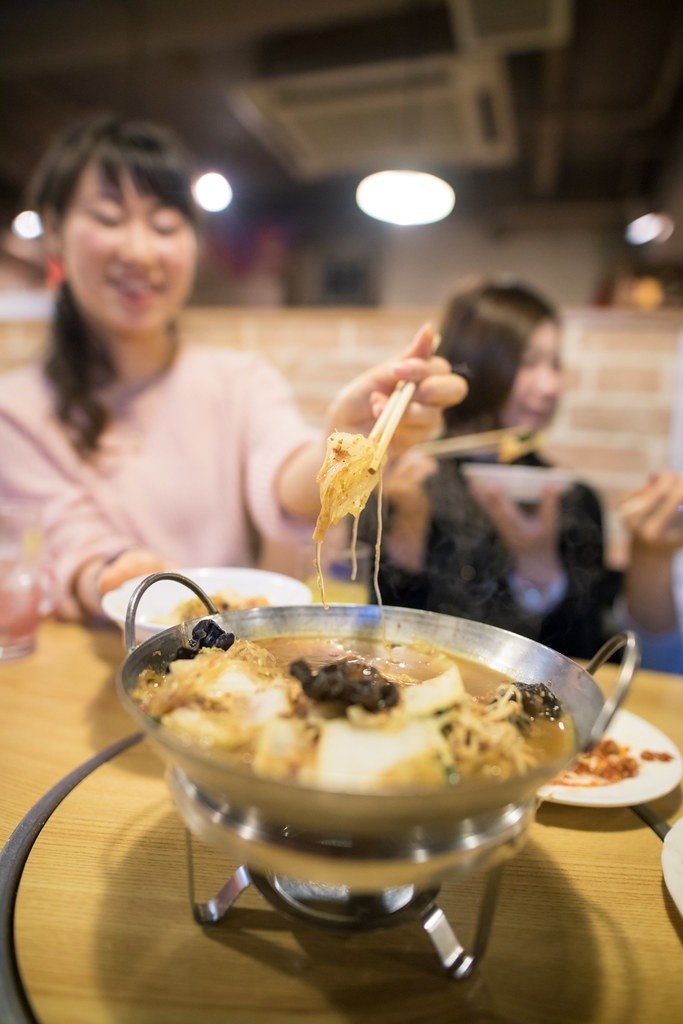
<box><xmin>0</xmin><ymin>618</ymin><xmax>683</xmax><ymax>1024</ymax></box>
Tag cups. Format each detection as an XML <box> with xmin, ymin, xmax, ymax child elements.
<box><xmin>0</xmin><ymin>498</ymin><xmax>44</xmax><ymax>660</ymax></box>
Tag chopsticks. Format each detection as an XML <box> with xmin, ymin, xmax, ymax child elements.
<box><xmin>365</xmin><ymin>333</ymin><xmax>440</xmax><ymax>475</ymax></box>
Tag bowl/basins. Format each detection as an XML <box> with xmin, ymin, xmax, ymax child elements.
<box><xmin>102</xmin><ymin>567</ymin><xmax>313</xmax><ymax>645</ymax></box>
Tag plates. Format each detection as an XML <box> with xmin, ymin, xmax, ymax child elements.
<box><xmin>536</xmin><ymin>708</ymin><xmax>683</xmax><ymax>807</ymax></box>
<box><xmin>661</xmin><ymin>816</ymin><xmax>683</xmax><ymax>918</ymax></box>
<box><xmin>462</xmin><ymin>464</ymin><xmax>575</xmax><ymax>504</ymax></box>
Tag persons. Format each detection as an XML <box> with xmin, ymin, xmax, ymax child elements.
<box><xmin>0</xmin><ymin>109</ymin><xmax>467</xmax><ymax>621</ymax></box>
<box><xmin>369</xmin><ymin>279</ymin><xmax>634</xmax><ymax>665</ymax></box>
<box><xmin>602</xmin><ymin>460</ymin><xmax>683</xmax><ymax>674</ymax></box>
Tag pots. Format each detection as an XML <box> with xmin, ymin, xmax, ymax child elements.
<box><xmin>116</xmin><ymin>570</ymin><xmax>640</xmax><ymax>843</ymax></box>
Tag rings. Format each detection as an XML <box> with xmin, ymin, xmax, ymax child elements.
<box><xmin>676</xmin><ymin>504</ymin><xmax>683</xmax><ymax>511</ymax></box>
